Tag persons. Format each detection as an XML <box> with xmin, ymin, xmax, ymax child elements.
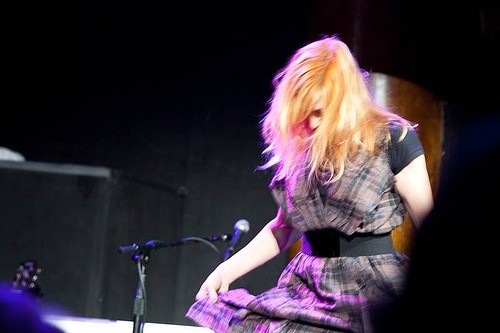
<box><xmin>185</xmin><ymin>37</ymin><xmax>434</xmax><ymax>333</ymax></box>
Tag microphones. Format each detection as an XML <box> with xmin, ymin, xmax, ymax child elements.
<box><xmin>223</xmin><ymin>219</ymin><xmax>249</xmax><ymax>261</ymax></box>
